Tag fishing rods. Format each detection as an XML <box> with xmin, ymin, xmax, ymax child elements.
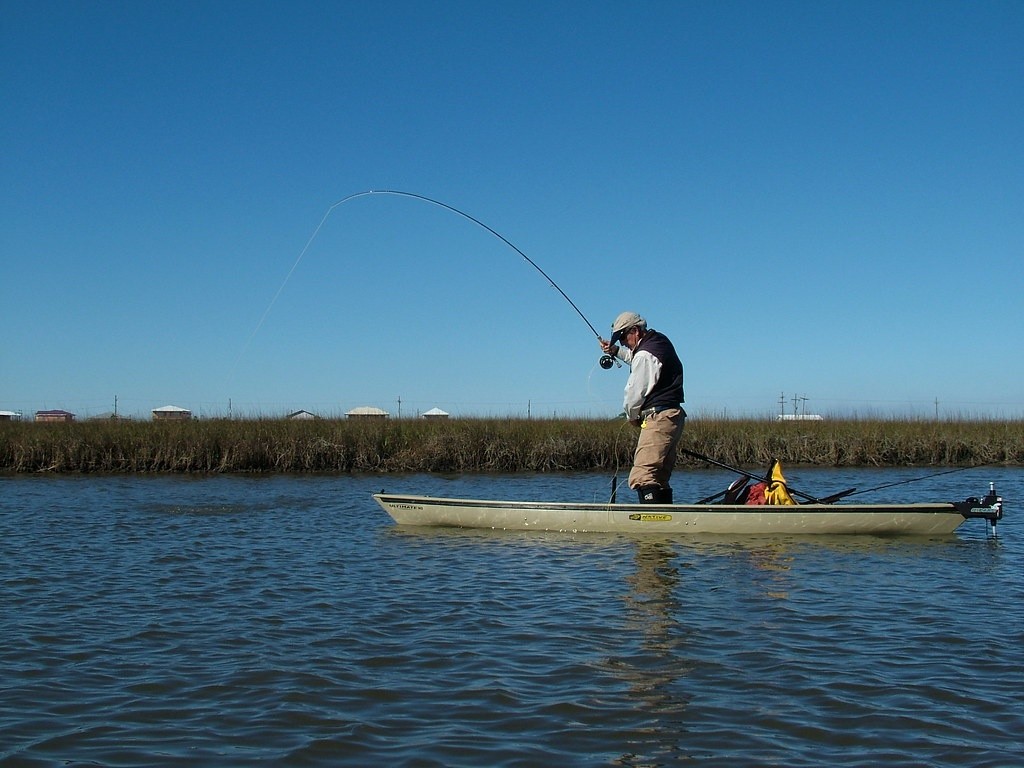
<box><xmin>332</xmin><ymin>190</ymin><xmax>622</xmax><ymax>367</ymax></box>
<box><xmin>826</xmin><ymin>455</ymin><xmax>1024</xmax><ymax>504</ymax></box>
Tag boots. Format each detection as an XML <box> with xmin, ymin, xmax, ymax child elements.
<box><xmin>636</xmin><ymin>484</ymin><xmax>661</xmax><ymax>504</ymax></box>
<box><xmin>661</xmin><ymin>488</ymin><xmax>673</xmax><ymax>503</ymax></box>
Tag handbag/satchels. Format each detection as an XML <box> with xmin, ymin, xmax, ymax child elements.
<box><xmin>764</xmin><ymin>457</ymin><xmax>801</xmax><ymax>506</ymax></box>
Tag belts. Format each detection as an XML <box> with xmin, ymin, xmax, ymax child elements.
<box><xmin>640</xmin><ymin>404</ymin><xmax>669</xmax><ymax>418</ymax></box>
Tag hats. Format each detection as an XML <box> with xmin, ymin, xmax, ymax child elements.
<box><xmin>609</xmin><ymin>312</ymin><xmax>647</xmax><ymax>348</ymax></box>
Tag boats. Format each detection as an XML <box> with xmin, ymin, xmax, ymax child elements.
<box><xmin>371</xmin><ymin>481</ymin><xmax>1003</xmax><ymax>538</ymax></box>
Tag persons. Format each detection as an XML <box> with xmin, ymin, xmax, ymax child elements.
<box><xmin>598</xmin><ymin>311</ymin><xmax>687</xmax><ymax>505</ymax></box>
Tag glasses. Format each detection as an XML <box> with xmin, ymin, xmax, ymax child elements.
<box><xmin>620</xmin><ymin>328</ymin><xmax>632</xmax><ymax>340</ymax></box>
